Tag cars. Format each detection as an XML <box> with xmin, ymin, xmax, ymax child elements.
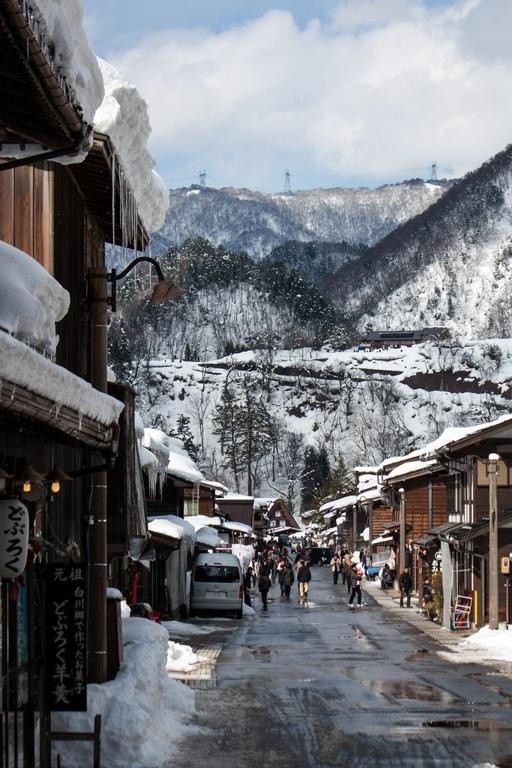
<box><xmin>279</xmin><ymin>534</ymin><xmax>292</xmax><ymax>548</ymax></box>
<box><xmin>365</xmin><ymin>566</ymin><xmax>381</xmax><ymax>581</ymax></box>
<box><xmin>291</xmin><ymin>539</ymin><xmax>303</xmax><ymax>552</ymax></box>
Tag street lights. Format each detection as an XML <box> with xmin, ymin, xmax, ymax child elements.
<box><xmin>381</xmin><ymin>485</ymin><xmax>405</xmax><ymax>574</ymax></box>
<box><xmin>89</xmin><ymin>256</ymin><xmax>186</xmax><ymax>681</ymax></box>
<box><xmin>462</xmin><ymin>454</ymin><xmax>499</xmax><ymax>629</ymax></box>
<box><xmin>357</xmin><ymin>495</ymin><xmax>374</xmax><ymax>552</ymax></box>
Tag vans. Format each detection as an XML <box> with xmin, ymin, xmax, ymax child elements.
<box><xmin>301</xmin><ymin>548</ymin><xmax>334</xmax><ymax>567</ymax></box>
<box><xmin>190</xmin><ymin>553</ymin><xmax>244</xmax><ymax>619</ymax></box>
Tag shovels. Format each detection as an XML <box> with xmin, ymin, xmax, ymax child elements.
<box><xmin>470</xmin><ymin>590</ymin><xmax>479</xmax><ymax>633</ymax></box>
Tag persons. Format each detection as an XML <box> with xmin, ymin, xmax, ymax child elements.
<box><xmin>257</xmin><ymin>570</ymin><xmax>272</xmax><ymax>613</ymax></box>
<box><xmin>247</xmin><ymin>532</ymin><xmax>354</xmax><ymax>594</ymax></box>
<box><xmin>296</xmin><ymin>561</ymin><xmax>312</xmax><ymax>599</ymax></box>
<box><xmin>356</xmin><ymin>566</ymin><xmax>363</xmax><ymax>585</ymax></box>
<box><xmin>279</xmin><ymin>564</ymin><xmax>287</xmax><ymax>596</ymax></box>
<box><xmin>243</xmin><ymin>566</ymin><xmax>253</xmax><ymax>607</ymax></box>
<box><xmin>379</xmin><ymin>563</ymin><xmax>395</xmax><ymax>590</ymax></box>
<box><xmin>281</xmin><ymin>562</ymin><xmax>295</xmax><ymax>598</ymax></box>
<box><xmin>347</xmin><ymin>562</ymin><xmax>365</xmax><ymax>608</ymax></box>
<box><xmin>398</xmin><ymin>566</ymin><xmax>413</xmax><ymax>609</ymax></box>
<box><xmin>358</xmin><ymin>547</ymin><xmax>373</xmax><ymax>569</ymax></box>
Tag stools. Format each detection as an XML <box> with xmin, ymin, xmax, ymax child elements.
<box><xmin>386</xmin><ymin>580</ymin><xmax>394</xmax><ymax>589</ymax></box>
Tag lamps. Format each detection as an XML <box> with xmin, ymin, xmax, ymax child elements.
<box><xmin>41</xmin><ymin>425</ymin><xmax>73</xmax><ymax>493</ymax></box>
<box><xmin>82</xmin><ymin>257</ymin><xmax>181</xmax><ymax>313</ymax></box>
<box><xmin>12</xmin><ymin>414</ymin><xmax>47</xmax><ymax>493</ymax></box>
